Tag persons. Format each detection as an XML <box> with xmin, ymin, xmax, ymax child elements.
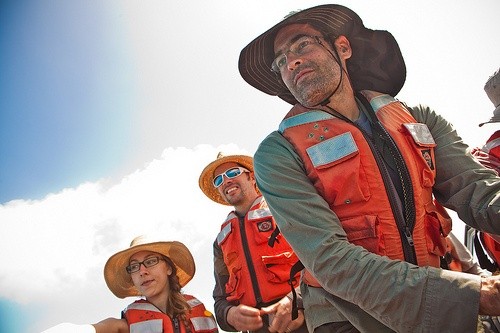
<box><xmin>239</xmin><ymin>4</ymin><xmax>500</xmax><ymax>333</ymax></box>
<box><xmin>467</xmin><ymin>67</ymin><xmax>500</xmax><ymax>275</ymax></box>
<box><xmin>197</xmin><ymin>148</ymin><xmax>307</xmax><ymax>333</ymax></box>
<box><xmin>39</xmin><ymin>235</ymin><xmax>219</xmax><ymax>333</ymax></box>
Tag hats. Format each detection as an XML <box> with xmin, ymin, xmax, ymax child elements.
<box><xmin>200</xmin><ymin>152</ymin><xmax>258</xmax><ymax>206</ymax></box>
<box><xmin>479</xmin><ymin>67</ymin><xmax>500</xmax><ymax>127</ymax></box>
<box><xmin>238</xmin><ymin>4</ymin><xmax>406</xmax><ymax>105</ymax></box>
<box><xmin>105</xmin><ymin>233</ymin><xmax>196</xmax><ymax>298</ymax></box>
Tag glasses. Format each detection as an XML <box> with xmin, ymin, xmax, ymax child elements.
<box><xmin>212</xmin><ymin>167</ymin><xmax>250</xmax><ymax>188</ymax></box>
<box><xmin>270</xmin><ymin>35</ymin><xmax>313</xmax><ymax>72</ymax></box>
<box><xmin>126</xmin><ymin>256</ymin><xmax>167</xmax><ymax>274</ymax></box>
